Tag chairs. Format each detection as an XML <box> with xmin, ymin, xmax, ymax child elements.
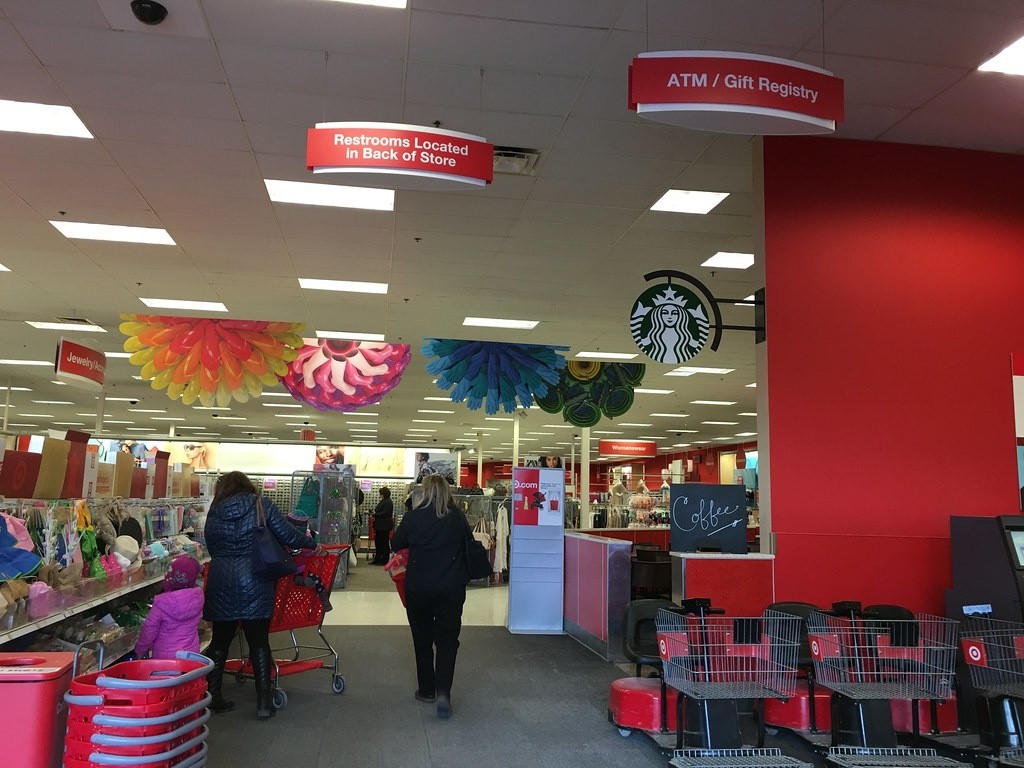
<box><xmin>609</xmin><ymin>596</ymin><xmax>686</xmax><ymax>735</ymax></box>
<box><xmin>631</xmin><ymin>560</ymin><xmax>671</xmax><ymax>599</ymax></box>
<box><xmin>861</xmin><ymin>604</ymin><xmax>959</xmax><ymax>737</ymax></box>
<box><xmin>763</xmin><ymin>601</ymin><xmax>833</xmax><ymax>736</ymax></box>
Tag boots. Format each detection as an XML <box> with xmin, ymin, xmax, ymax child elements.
<box><xmin>206</xmin><ymin>650</ymin><xmax>234</xmax><ymax>712</ymax></box>
<box><xmin>250</xmin><ymin>648</ymin><xmax>275</xmax><ymax>717</ymax></box>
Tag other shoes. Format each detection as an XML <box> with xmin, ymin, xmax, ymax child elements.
<box><xmin>368</xmin><ymin>560</ymin><xmax>387</xmax><ymax>566</ymax></box>
<box><xmin>415</xmin><ymin>689</ymin><xmax>436</xmax><ymax>702</ymax></box>
<box><xmin>437</xmin><ymin>695</ymin><xmax>451</xmax><ymax>719</ymax></box>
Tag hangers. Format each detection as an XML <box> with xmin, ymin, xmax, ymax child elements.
<box><xmin>500</xmin><ymin>502</ymin><xmax>507</xmax><ymax>511</ymax></box>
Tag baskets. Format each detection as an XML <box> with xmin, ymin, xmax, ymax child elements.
<box><xmin>61</xmin><ymin>640</ymin><xmax>214</xmax><ymax>768</ymax></box>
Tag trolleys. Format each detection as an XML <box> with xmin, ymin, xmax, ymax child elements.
<box><xmin>806</xmin><ymin>606</ymin><xmax>974</xmax><ymax>768</ymax></box>
<box><xmin>199</xmin><ymin>544</ymin><xmax>351</xmax><ymax>710</ymax></box>
<box><xmin>366</xmin><ymin>514</ymin><xmax>396</xmax><ymax>561</ymax></box>
<box><xmin>959</xmin><ymin>615</ymin><xmax>1024</xmax><ymax>768</ymax></box>
<box><xmin>655</xmin><ymin>596</ymin><xmax>815</xmax><ymax>768</ymax></box>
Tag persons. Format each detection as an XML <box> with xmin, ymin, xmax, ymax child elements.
<box><xmin>329</xmin><ymin>464</ymin><xmax>339</xmax><ymax>471</ymax></box>
<box><xmin>184</xmin><ymin>443</ymin><xmax>208</xmax><ymax>469</ymax></box>
<box><xmin>612</xmin><ymin>475</ymin><xmax>628</xmax><ymax>497</ymax></box>
<box><xmin>538</xmin><ymin>456</ymin><xmax>562</xmax><ymax>468</ymax></box>
<box><xmin>661</xmin><ymin>477</ymin><xmax>672</xmax><ymax>490</ymax></box>
<box><xmin>121</xmin><ymin>440</ymin><xmax>146</xmax><ymax>468</ymax></box>
<box><xmin>343</xmin><ymin>464</ymin><xmax>355</xmax><ymax>475</ymax></box>
<box><xmin>369</xmin><ymin>487</ymin><xmax>394</xmax><ymax>565</ymax></box>
<box><xmin>417</xmin><ymin>453</ymin><xmax>434</xmax><ymax>477</ymax></box>
<box><xmin>390</xmin><ymin>474</ymin><xmax>474</xmax><ymax>718</ymax></box>
<box><xmin>637</xmin><ymin>484</ymin><xmax>650</xmax><ymax>497</ymax></box>
<box><xmin>203</xmin><ymin>472</ymin><xmax>317</xmax><ymax>716</ymax></box>
<box><xmin>135</xmin><ymin>557</ymin><xmax>204</xmax><ymax>660</ymax></box>
<box><xmin>316</xmin><ymin>445</ymin><xmax>343</xmax><ymax>464</ymax></box>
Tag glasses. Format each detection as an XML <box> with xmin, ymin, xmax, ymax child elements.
<box><xmin>184</xmin><ymin>445</ymin><xmax>202</xmax><ymax>451</ymax></box>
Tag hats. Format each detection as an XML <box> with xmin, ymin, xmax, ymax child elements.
<box><xmin>162</xmin><ymin>556</ymin><xmax>199</xmax><ymax>591</ymax></box>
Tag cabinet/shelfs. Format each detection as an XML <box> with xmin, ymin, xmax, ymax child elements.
<box><xmin>0</xmin><ymin>557</ymin><xmax>212</xmax><ymax>674</ymax></box>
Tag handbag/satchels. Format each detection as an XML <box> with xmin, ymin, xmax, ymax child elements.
<box><xmin>472</xmin><ymin>518</ymin><xmax>493</xmax><ymax>550</ymax></box>
<box><xmin>458</xmin><ymin>484</ymin><xmax>469</xmax><ymax>495</ymax></box>
<box><xmin>385</xmin><ymin>548</ymin><xmax>409</xmax><ymax>607</ymax></box>
<box><xmin>252</xmin><ymin>497</ymin><xmax>297</xmax><ymax>580</ymax></box>
<box><xmin>470</xmin><ymin>483</ymin><xmax>483</xmax><ymax>495</ymax></box>
<box><xmin>494</xmin><ymin>484</ymin><xmax>506</xmax><ymax>496</ymax></box>
<box><xmin>296</xmin><ymin>477</ymin><xmax>319</xmax><ymax>517</ymax></box>
<box><xmin>458</xmin><ymin>507</ymin><xmax>493</xmax><ymax>580</ymax></box>
